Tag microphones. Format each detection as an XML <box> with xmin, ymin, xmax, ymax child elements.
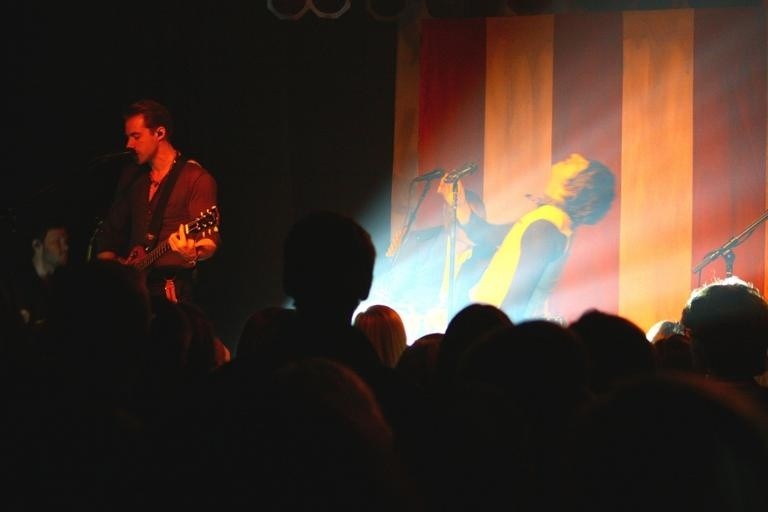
<box><xmin>102</xmin><ymin>150</ymin><xmax>134</xmax><ymax>160</ymax></box>
<box><xmin>452</xmin><ymin>163</ymin><xmax>477</xmax><ymax>180</ymax></box>
<box><xmin>416</xmin><ymin>169</ymin><xmax>443</xmax><ymax>181</ymax></box>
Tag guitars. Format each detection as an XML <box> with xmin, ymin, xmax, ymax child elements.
<box><xmin>124</xmin><ymin>205</ymin><xmax>219</xmax><ymax>296</ymax></box>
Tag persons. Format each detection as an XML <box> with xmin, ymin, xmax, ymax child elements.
<box><xmin>1</xmin><ymin>205</ymin><xmax>767</xmax><ymax>512</ymax></box>
<box><xmin>90</xmin><ymin>95</ymin><xmax>219</xmax><ymax>322</ymax></box>
<box><xmin>437</xmin><ymin>150</ymin><xmax>617</xmax><ymax>328</ymax></box>
<box><xmin>437</xmin><ymin>187</ymin><xmax>487</xmax><ymax>330</ymax></box>
